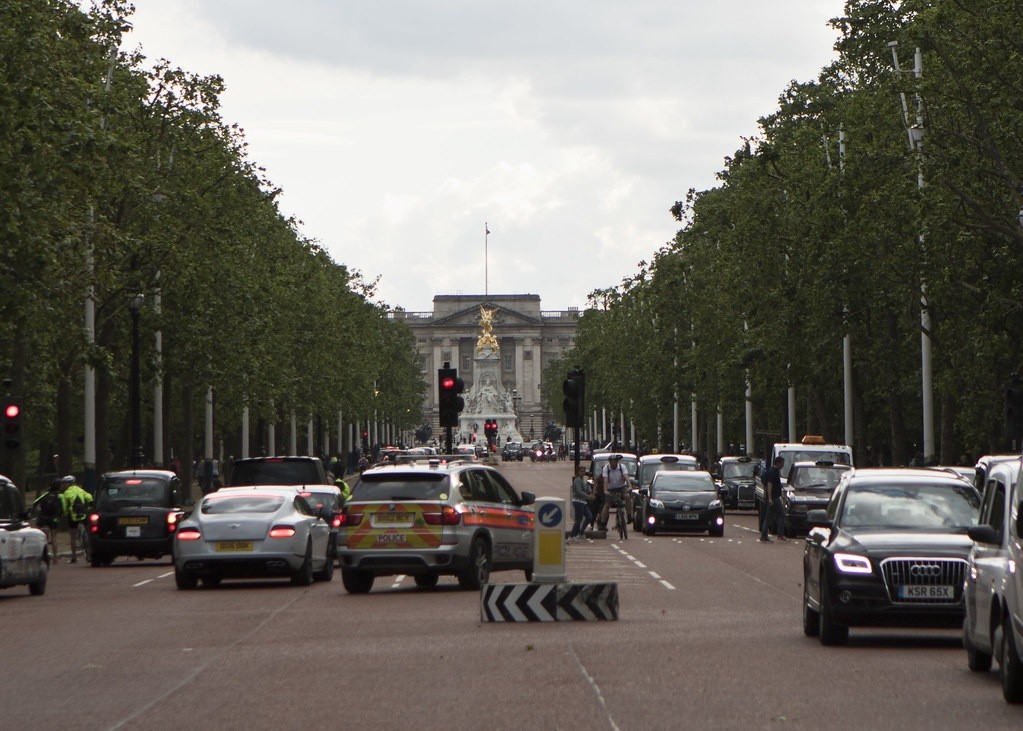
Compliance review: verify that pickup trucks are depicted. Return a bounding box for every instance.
[754,435,855,521]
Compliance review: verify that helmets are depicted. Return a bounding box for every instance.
[51,478,63,486]
[609,455,618,462]
[62,474,77,483]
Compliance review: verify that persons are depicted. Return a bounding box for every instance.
[169,453,237,492]
[323,445,380,479]
[40,475,93,564]
[428,448,458,459]
[558,444,566,461]
[572,455,635,535]
[825,472,836,487]
[760,457,791,542]
[796,468,810,486]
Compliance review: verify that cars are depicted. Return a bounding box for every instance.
[802,466,987,648]
[171,485,333,588]
[83,469,183,570]
[230,455,328,486]
[591,453,697,505]
[0,474,53,593]
[781,459,851,536]
[719,457,769,507]
[643,469,726,534]
[374,441,593,464]
[338,458,541,588]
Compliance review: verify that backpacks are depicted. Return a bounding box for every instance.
[40,492,59,517]
[66,487,85,514]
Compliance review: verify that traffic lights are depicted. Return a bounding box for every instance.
[491,420,498,437]
[563,370,584,427]
[484,419,491,436]
[1,393,22,450]
[438,367,465,427]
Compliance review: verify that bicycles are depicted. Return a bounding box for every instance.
[611,494,631,539]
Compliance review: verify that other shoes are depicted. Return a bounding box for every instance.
[760,538,773,543]
[567,535,591,542]
[627,515,635,524]
[777,536,791,542]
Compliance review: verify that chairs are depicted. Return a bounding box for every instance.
[853,502,881,526]
